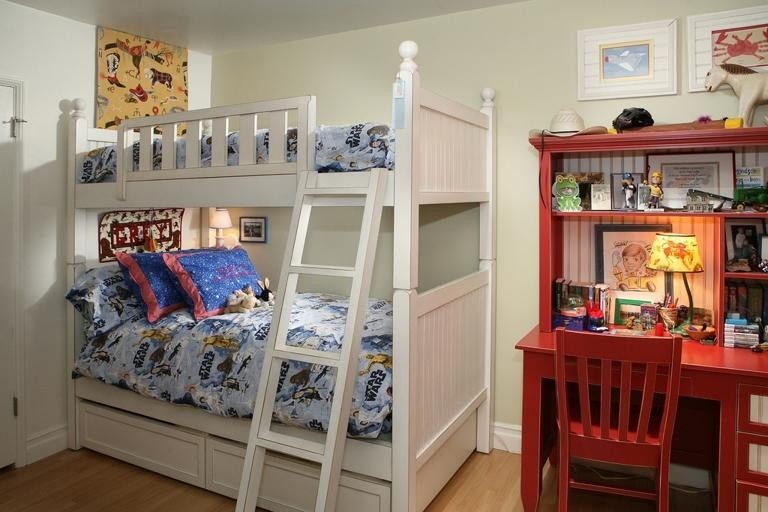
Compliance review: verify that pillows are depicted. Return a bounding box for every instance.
[113,251,190,324]
[161,243,264,322]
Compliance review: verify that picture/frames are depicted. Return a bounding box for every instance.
[725,217,766,264]
[576,18,678,102]
[239,216,267,243]
[686,4,767,94]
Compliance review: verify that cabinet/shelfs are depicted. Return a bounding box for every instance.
[513,128,766,511]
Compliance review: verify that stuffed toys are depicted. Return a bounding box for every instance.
[223,284,261,314]
[253,276,275,307]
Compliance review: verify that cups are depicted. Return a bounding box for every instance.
[660,307,678,329]
[656,323,663,337]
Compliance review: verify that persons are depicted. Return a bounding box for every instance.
[643,170,664,209]
[620,171,637,210]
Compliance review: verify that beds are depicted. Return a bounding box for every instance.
[65,41,497,512]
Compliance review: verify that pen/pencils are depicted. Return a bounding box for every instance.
[658,293,679,308]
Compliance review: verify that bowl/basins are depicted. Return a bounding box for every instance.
[682,325,716,340]
[550,109,584,132]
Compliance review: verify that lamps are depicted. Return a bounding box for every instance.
[644,231,705,337]
[208,209,232,247]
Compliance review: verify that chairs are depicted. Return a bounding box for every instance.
[554,325,682,512]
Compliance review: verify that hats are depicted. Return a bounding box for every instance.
[529,110,608,139]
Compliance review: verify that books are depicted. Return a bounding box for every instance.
[554,277,611,326]
[723,312,760,349]
